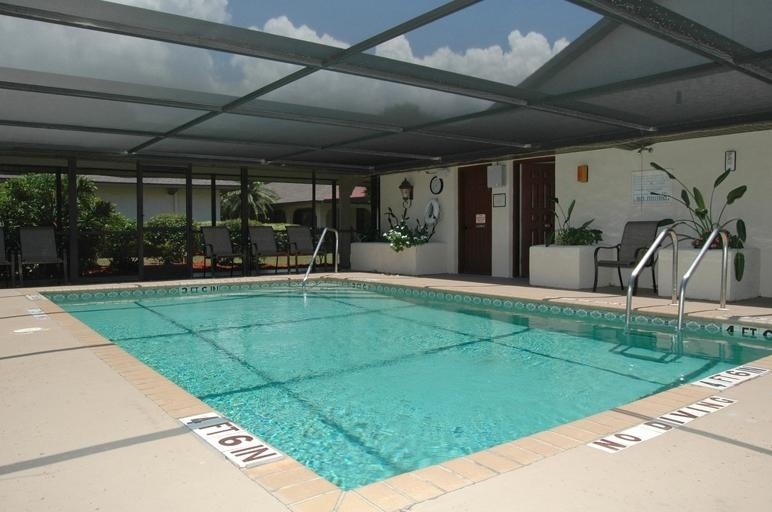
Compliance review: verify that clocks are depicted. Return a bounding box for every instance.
[429,175,443,195]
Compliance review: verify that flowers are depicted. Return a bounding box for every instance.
[382,207,437,253]
[646,150,752,283]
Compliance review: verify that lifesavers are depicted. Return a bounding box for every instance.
[424,199,439,225]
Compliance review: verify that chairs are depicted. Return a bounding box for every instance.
[0,225,70,289]
[199,223,327,279]
[592,218,668,296]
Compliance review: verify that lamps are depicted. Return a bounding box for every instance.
[398,178,414,200]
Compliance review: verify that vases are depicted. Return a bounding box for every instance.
[350,240,446,277]
[656,245,759,302]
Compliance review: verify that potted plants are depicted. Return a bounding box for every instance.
[526,193,612,292]
